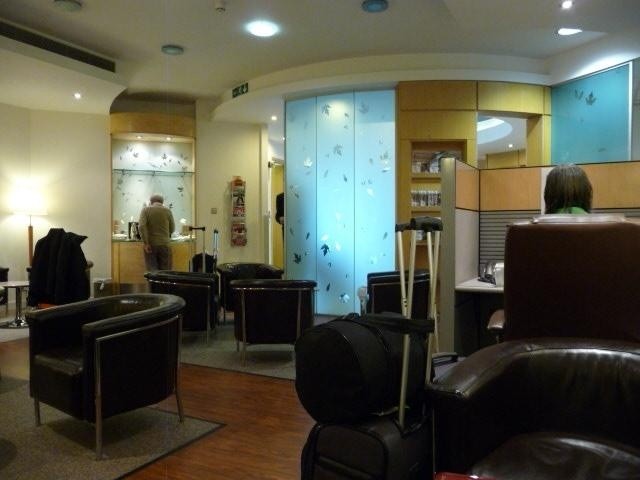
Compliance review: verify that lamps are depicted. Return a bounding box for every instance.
[13,173,48,267]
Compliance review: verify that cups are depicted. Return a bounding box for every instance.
[478,262,495,281]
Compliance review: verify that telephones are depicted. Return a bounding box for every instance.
[481,261,495,283]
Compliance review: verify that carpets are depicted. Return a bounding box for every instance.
[178,312,338,383]
[0,374,227,480]
[0,321,28,345]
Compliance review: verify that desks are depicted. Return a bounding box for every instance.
[1,280,32,328]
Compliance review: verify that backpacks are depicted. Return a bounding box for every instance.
[27,228,90,306]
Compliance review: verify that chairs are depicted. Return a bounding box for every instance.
[24,293,186,462]
[488,217,639,342]
[216,262,284,325]
[424,334,640,480]
[232,278,317,368]
[145,273,219,344]
[1,267,11,314]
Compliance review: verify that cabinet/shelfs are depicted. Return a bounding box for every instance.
[111,112,196,286]
[480,83,552,115]
[398,80,477,316]
[524,117,553,166]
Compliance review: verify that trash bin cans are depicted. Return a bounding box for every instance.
[94,278,112,298]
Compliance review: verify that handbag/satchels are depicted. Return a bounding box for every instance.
[295,311,428,425]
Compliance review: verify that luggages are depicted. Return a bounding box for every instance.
[302,216,441,479]
[189,230,218,273]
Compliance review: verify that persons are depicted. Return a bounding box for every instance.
[542,160,592,214]
[138,195,175,271]
[275,179,284,242]
[232,176,246,246]
